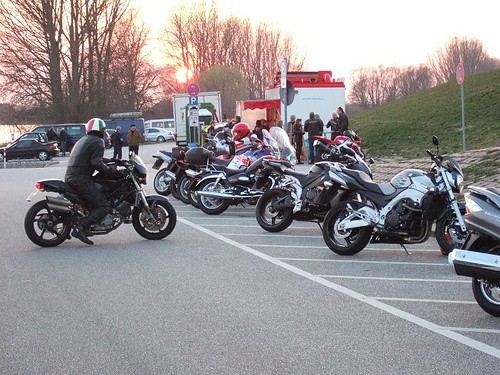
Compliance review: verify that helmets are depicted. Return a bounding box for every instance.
[86,118,106,139]
[232,122,250,141]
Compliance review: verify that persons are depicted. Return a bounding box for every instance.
[126,124,142,159]
[46,128,57,142]
[111,126,126,159]
[65,118,114,245]
[326,107,348,140]
[226,116,241,129]
[60,128,67,156]
[208,122,215,136]
[304,112,323,164]
[254,119,270,140]
[288,115,306,164]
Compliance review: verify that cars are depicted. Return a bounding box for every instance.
[145,127,174,143]
[0,132,60,163]
[104,128,124,149]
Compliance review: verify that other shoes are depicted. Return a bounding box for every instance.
[308,161,314,164]
[71,228,94,245]
[298,162,304,164]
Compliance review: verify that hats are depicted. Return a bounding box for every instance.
[291,115,295,119]
[116,126,121,131]
[131,124,136,126]
[314,114,319,116]
[310,112,314,117]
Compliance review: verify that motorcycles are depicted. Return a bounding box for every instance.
[23,150,178,248]
[149,129,472,256]
[447,184,500,318]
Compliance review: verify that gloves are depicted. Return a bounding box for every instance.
[109,168,124,179]
[110,157,123,166]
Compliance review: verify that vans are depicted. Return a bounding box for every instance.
[30,123,88,147]
[143,118,177,135]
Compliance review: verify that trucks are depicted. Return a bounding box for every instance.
[103,112,145,143]
